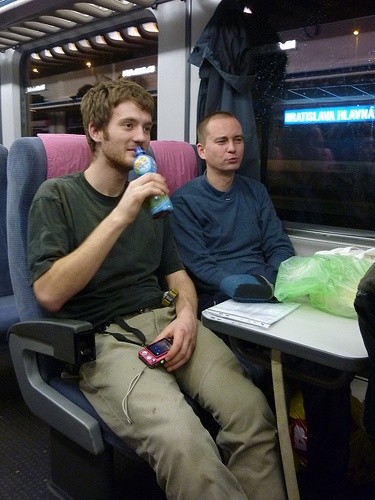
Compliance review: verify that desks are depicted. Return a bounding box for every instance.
[201,300,368,500]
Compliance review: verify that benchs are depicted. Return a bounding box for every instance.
[7,134,202,500]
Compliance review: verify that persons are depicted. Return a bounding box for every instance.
[27,80,290,500]
[168,112,353,500]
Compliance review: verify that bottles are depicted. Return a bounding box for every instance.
[133,147,174,218]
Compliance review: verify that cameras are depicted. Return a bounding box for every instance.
[138,337,172,369]
